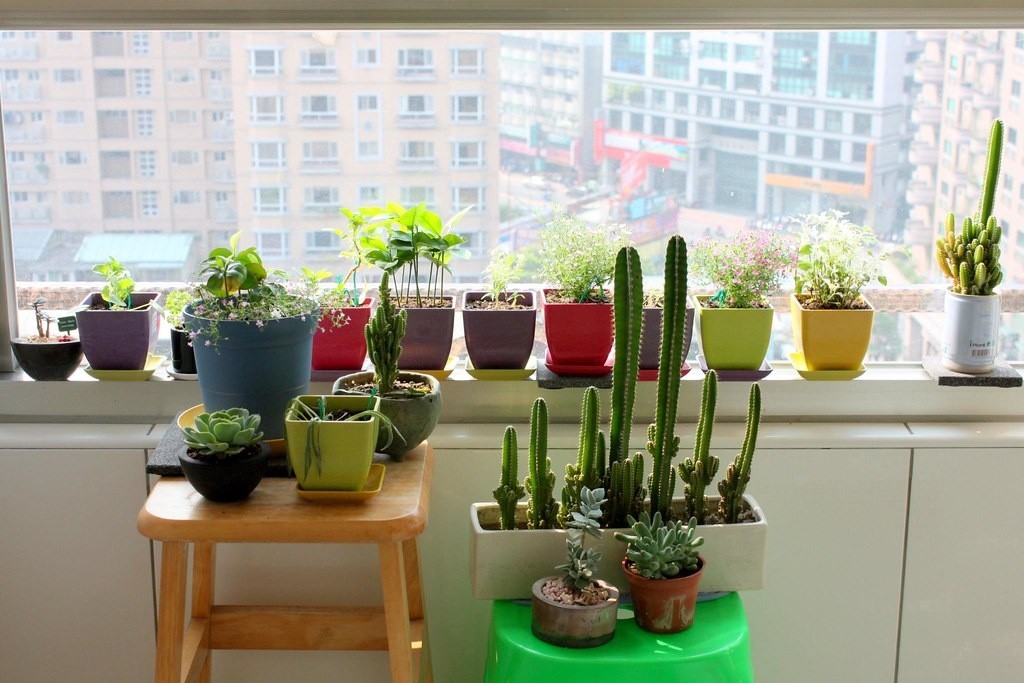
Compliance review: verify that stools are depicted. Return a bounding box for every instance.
[482,593,754,683]
[137,438,442,683]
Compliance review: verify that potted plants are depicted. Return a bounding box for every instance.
[609,280,697,373]
[10,298,83,383]
[465,240,768,598]
[360,197,470,372]
[283,388,405,491]
[332,268,442,456]
[618,506,712,640]
[75,256,161,371]
[159,287,206,372]
[935,117,1004,372]
[462,246,536,370]
[176,407,270,504]
[529,470,615,648]
[298,197,384,371]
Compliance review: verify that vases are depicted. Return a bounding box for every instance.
[792,282,873,368]
[544,287,618,370]
[183,294,321,437]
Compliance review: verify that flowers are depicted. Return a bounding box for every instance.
[688,221,795,308]
[173,235,345,344]
[536,218,628,305]
[778,208,910,304]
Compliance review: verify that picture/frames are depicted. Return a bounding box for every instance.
[697,298,772,375]
